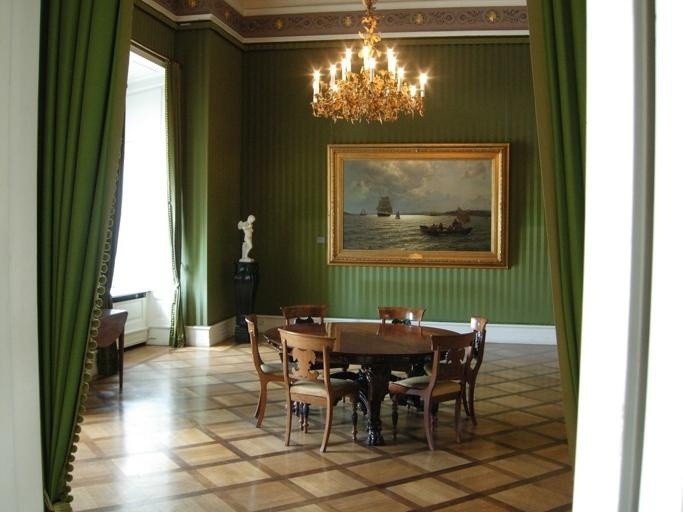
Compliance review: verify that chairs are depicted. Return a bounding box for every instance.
[245,314,304,430]
[279,304,349,403]
[378,306,426,408]
[424,315,489,425]
[388,330,477,451]
[277,327,360,453]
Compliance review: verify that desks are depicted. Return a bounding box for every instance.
[264,322,464,445]
[97,309,128,392]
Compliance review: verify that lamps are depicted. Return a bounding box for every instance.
[310,0,427,125]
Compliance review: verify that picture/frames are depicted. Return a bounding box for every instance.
[326,143,510,268]
